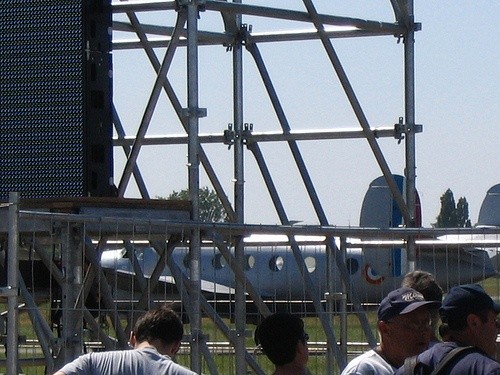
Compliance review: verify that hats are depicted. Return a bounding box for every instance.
[377,288,440,321]
[439,284,500,325]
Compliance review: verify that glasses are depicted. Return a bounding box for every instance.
[386,317,436,331]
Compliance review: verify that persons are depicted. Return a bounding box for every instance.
[51,307,201,375]
[340,270,500,375]
[252,313,318,375]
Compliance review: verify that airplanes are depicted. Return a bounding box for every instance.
[99,174,500,316]
